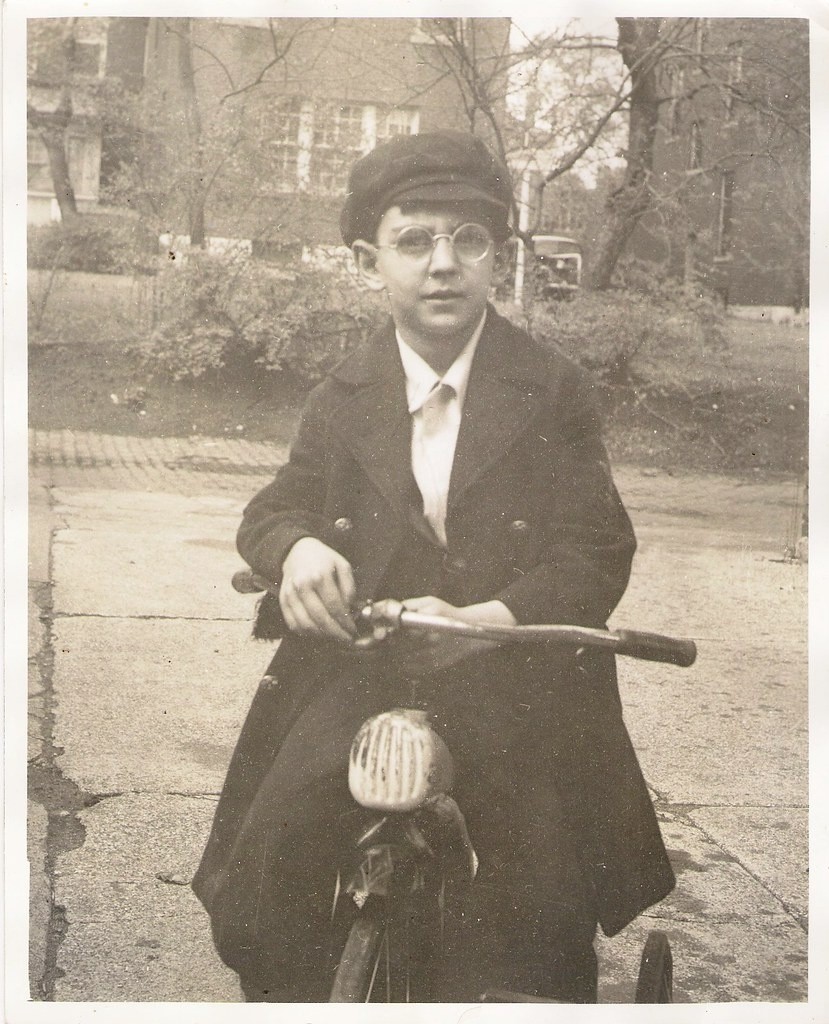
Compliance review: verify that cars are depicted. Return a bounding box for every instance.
[41,207,163,276]
[497,235,585,303]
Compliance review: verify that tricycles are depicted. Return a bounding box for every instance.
[232,569,698,1003]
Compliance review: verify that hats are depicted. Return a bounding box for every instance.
[339,129,512,248]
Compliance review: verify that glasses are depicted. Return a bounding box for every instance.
[371,222,497,265]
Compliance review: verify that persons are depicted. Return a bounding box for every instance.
[188,130,674,1003]
